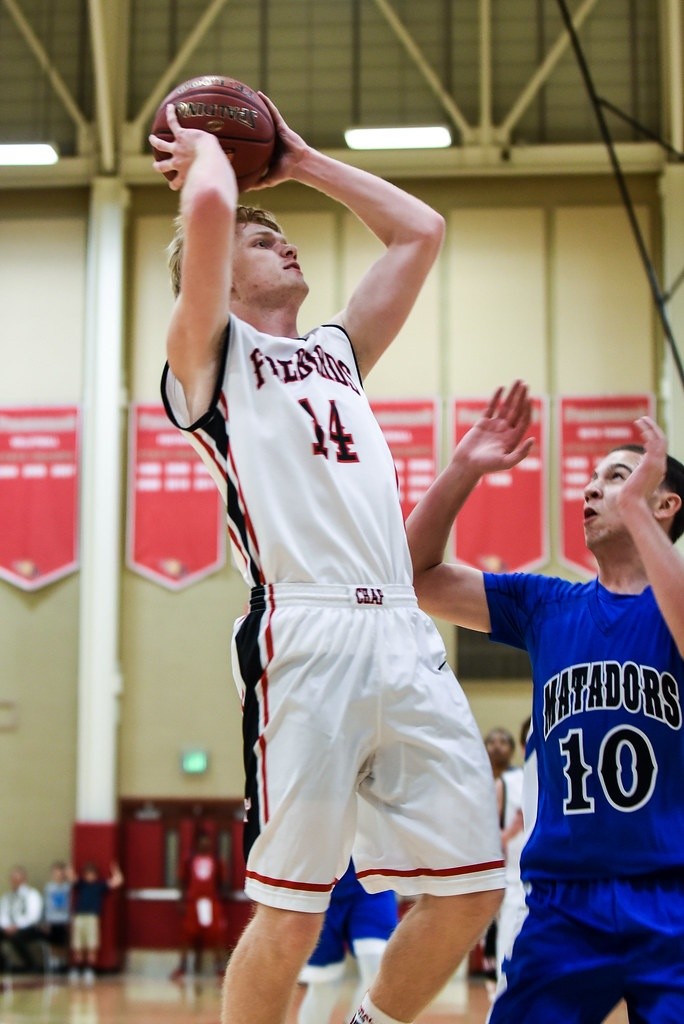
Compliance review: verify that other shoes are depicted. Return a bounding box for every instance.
[84,971,95,986]
[68,969,82,984]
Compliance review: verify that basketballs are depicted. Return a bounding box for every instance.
[151,77,279,196]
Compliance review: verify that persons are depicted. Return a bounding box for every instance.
[284,713,529,1024]
[165,818,231,979]
[64,861,123,985]
[402,377,684,1024]
[0,859,73,975]
[148,90,504,1024]
